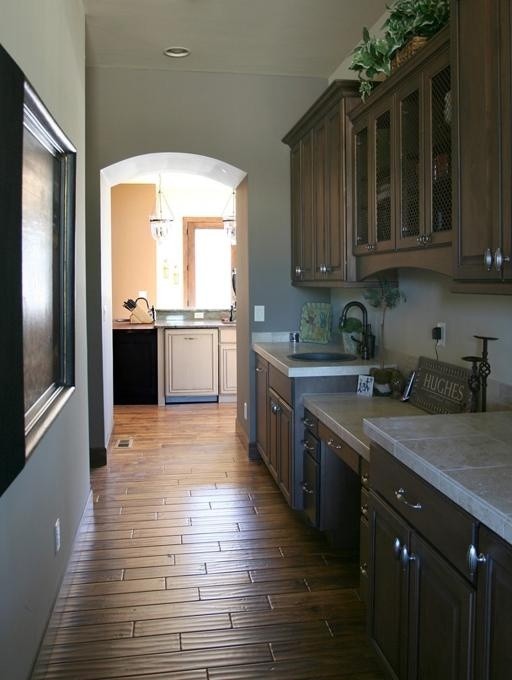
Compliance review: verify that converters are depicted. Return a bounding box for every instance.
[431,327,442,340]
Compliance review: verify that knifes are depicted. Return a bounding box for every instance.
[122,298,136,312]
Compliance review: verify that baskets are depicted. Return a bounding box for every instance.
[390,34,429,74]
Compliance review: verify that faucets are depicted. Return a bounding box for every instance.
[339,301,376,358]
[134,298,156,321]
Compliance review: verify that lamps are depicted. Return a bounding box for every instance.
[223,186,238,246]
[150,172,175,242]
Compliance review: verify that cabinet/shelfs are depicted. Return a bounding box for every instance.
[351,442,512,680]
[219,327,236,403]
[163,327,218,406]
[301,390,459,607]
[345,1,509,300]
[281,77,395,289]
[253,354,397,510]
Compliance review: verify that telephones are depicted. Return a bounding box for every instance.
[399,370,416,402]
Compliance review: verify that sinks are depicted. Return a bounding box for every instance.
[287,352,358,362]
[115,317,132,322]
